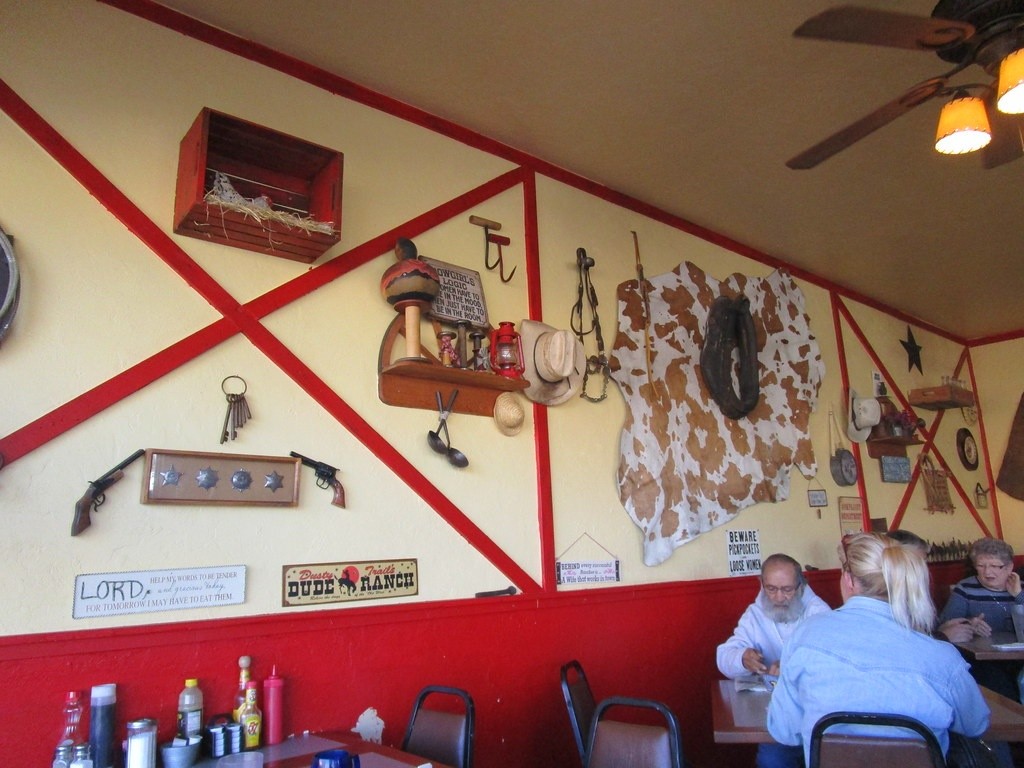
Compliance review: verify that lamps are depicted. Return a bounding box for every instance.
[934,87,992,155]
[489,322,526,378]
[996,47,1024,114]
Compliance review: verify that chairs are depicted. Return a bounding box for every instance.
[401,657,699,768]
[809,711,948,768]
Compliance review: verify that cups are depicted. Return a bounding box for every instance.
[1009,604,1024,645]
[310,749,361,768]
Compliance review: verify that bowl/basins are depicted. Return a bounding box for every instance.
[160,738,202,768]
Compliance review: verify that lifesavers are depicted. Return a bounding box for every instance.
[699,295,760,421]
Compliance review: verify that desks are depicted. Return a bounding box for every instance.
[157,729,459,768]
[710,679,1024,744]
[953,631,1024,661]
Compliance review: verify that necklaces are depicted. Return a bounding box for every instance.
[990,594,1006,611]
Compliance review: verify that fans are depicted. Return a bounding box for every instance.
[785,0,1024,171]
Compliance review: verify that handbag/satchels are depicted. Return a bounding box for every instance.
[947,732,998,768]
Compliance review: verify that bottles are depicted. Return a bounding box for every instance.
[262,664,286,746]
[233,656,254,727]
[225,722,244,754]
[207,725,227,758]
[216,750,264,768]
[178,678,203,742]
[940,375,968,391]
[52,691,95,768]
[126,717,158,768]
[88,683,118,768]
[239,681,263,751]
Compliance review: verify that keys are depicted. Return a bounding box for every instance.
[219,392,251,444]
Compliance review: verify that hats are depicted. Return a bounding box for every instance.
[848,387,880,443]
[494,392,526,436]
[517,319,586,405]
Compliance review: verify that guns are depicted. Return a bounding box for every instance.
[289,450,347,510]
[69,447,145,537]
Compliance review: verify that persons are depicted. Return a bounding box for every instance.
[937,536,1024,768]
[885,529,992,644]
[767,530,991,768]
[715,554,834,768]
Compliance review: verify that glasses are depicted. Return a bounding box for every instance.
[974,562,1008,573]
[762,579,801,595]
[842,533,859,586]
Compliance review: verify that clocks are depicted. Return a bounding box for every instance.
[956,428,978,471]
